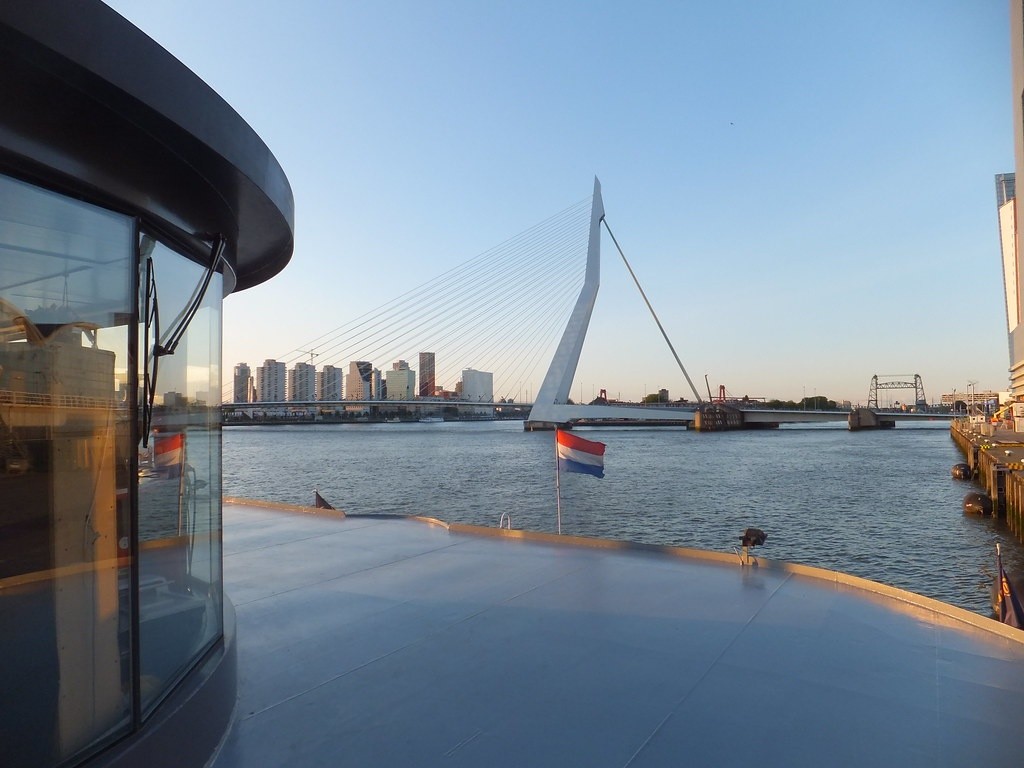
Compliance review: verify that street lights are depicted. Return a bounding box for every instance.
[953,388,958,420]
[966,383,972,415]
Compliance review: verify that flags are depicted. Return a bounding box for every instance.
[153,434,184,477]
[557,429,605,479]
[996,559,1024,629]
